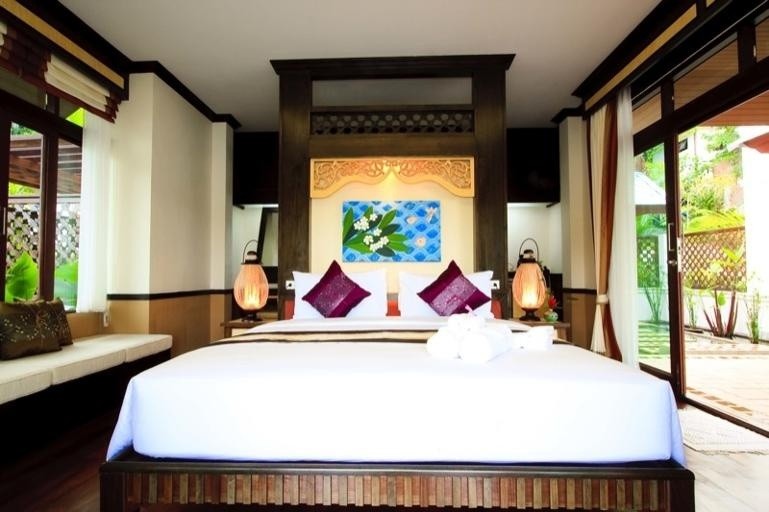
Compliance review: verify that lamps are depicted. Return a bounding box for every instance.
[233,239,270,322]
[512,237,549,321]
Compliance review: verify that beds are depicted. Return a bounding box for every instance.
[98,294,697,511]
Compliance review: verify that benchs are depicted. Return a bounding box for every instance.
[0,309,174,464]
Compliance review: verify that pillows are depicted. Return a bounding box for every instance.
[1,296,73,361]
[291,260,495,325]
